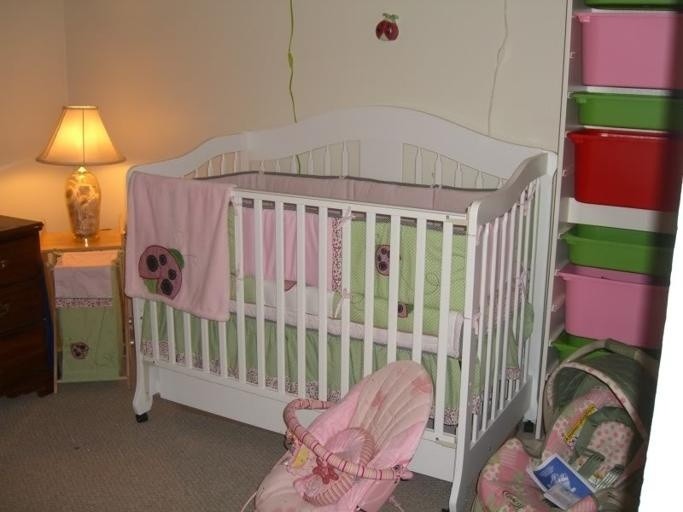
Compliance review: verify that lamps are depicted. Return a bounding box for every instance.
[36,105,124,244]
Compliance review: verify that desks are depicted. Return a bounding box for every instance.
[48,247,130,384]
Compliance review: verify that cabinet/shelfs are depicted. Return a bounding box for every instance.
[0,215,60,398]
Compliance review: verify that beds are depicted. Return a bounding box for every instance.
[126,105,559,512]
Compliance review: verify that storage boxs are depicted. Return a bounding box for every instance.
[563,197,678,234]
[563,124,682,211]
[553,262,669,353]
[570,83,681,131]
[572,0,682,90]
[563,226,673,279]
